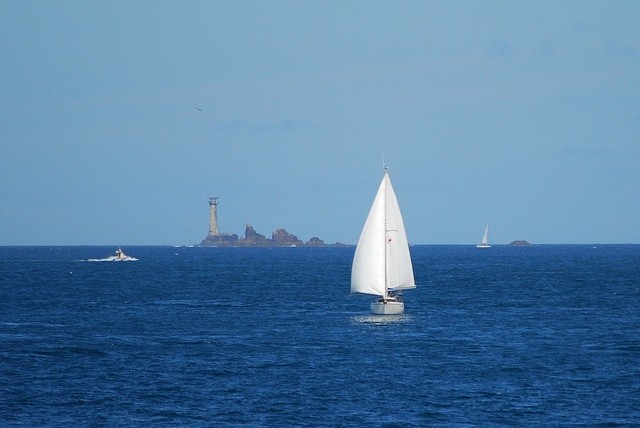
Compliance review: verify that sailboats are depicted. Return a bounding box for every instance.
[349,172,417,315]
[477,225,492,248]
